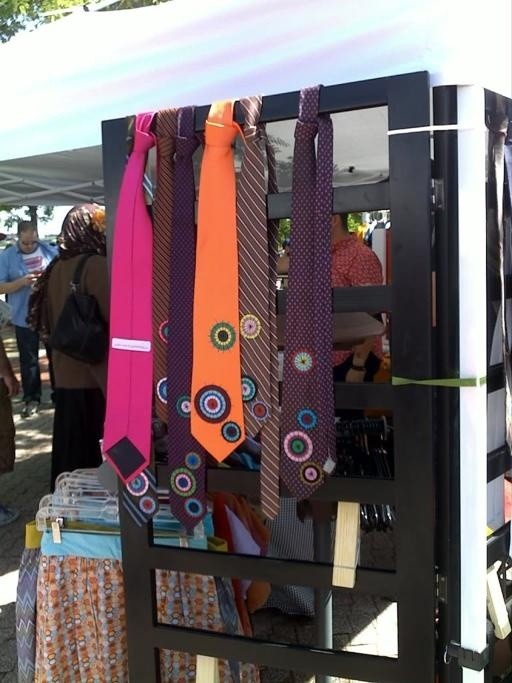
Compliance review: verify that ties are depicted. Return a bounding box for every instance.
[278,83,338,502]
[118,113,156,528]
[152,107,176,424]
[190,101,246,464]
[236,95,278,521]
[168,107,207,530]
[102,110,156,485]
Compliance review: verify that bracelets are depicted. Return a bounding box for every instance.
[350,364,366,372]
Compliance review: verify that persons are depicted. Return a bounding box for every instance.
[276,211,386,422]
[22,201,113,496]
[0,218,61,417]
[0,336,22,530]
[279,236,292,288]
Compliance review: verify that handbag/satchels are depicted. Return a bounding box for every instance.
[48,255,110,366]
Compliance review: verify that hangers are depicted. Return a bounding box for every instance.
[32,440,206,548]
[334,413,396,532]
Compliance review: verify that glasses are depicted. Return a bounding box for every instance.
[22,240,36,246]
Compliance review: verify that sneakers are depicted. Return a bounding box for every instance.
[0,503,22,527]
[20,399,41,418]
